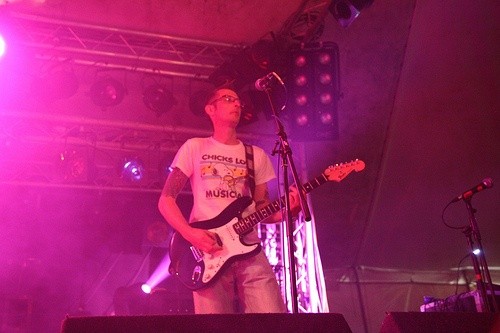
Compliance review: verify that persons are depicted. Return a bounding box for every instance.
[157,80,307,314]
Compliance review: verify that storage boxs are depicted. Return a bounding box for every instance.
[419,288,500,312]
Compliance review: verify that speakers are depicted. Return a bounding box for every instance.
[62,313,352,333]
[378,311,500,333]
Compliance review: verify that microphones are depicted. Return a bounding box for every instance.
[255,72,275,90]
[452,178,494,203]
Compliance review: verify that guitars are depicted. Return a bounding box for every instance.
[168,158,366,292]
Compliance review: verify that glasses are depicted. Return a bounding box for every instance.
[210,95,244,108]
